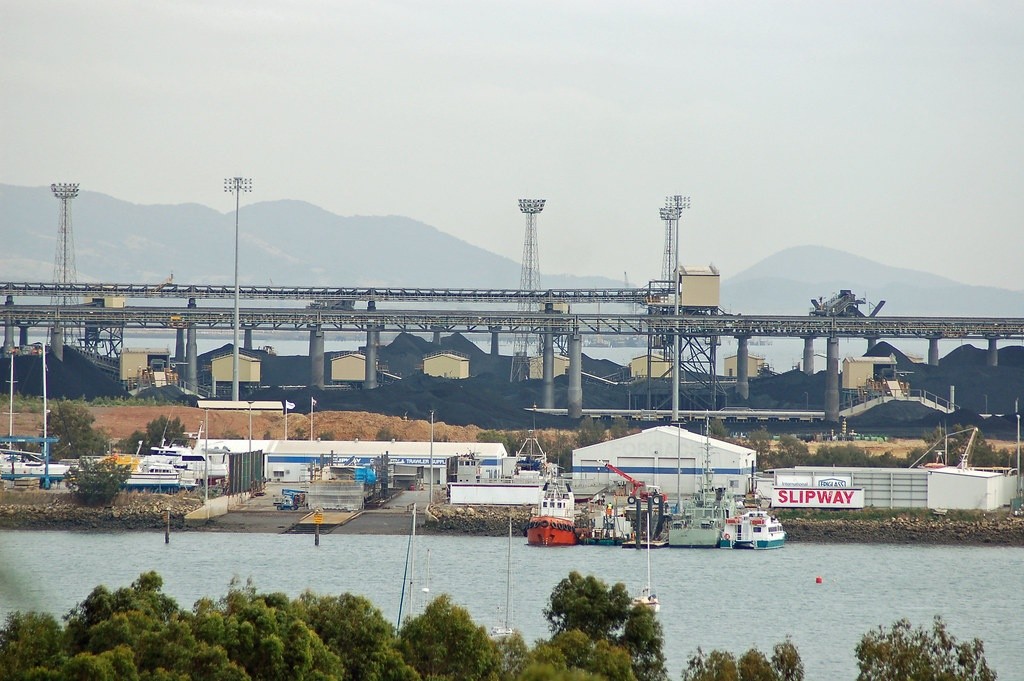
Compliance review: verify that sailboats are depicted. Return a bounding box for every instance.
[627,511,661,617]
[483,516,516,647]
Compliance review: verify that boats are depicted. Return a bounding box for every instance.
[836,367,959,429]
[523,468,582,545]
[0,421,230,494]
[574,469,788,550]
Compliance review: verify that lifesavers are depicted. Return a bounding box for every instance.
[724,534,730,540]
[541,520,549,527]
[568,525,572,531]
[526,521,529,529]
[534,521,539,528]
[558,522,564,530]
[529,522,534,528]
[550,521,557,529]
[564,523,568,530]
[522,526,527,537]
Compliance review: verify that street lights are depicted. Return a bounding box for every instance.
[663,195,693,423]
[518,196,546,323]
[803,391,809,413]
[223,176,254,400]
[50,178,81,346]
[982,393,989,414]
[660,207,683,302]
